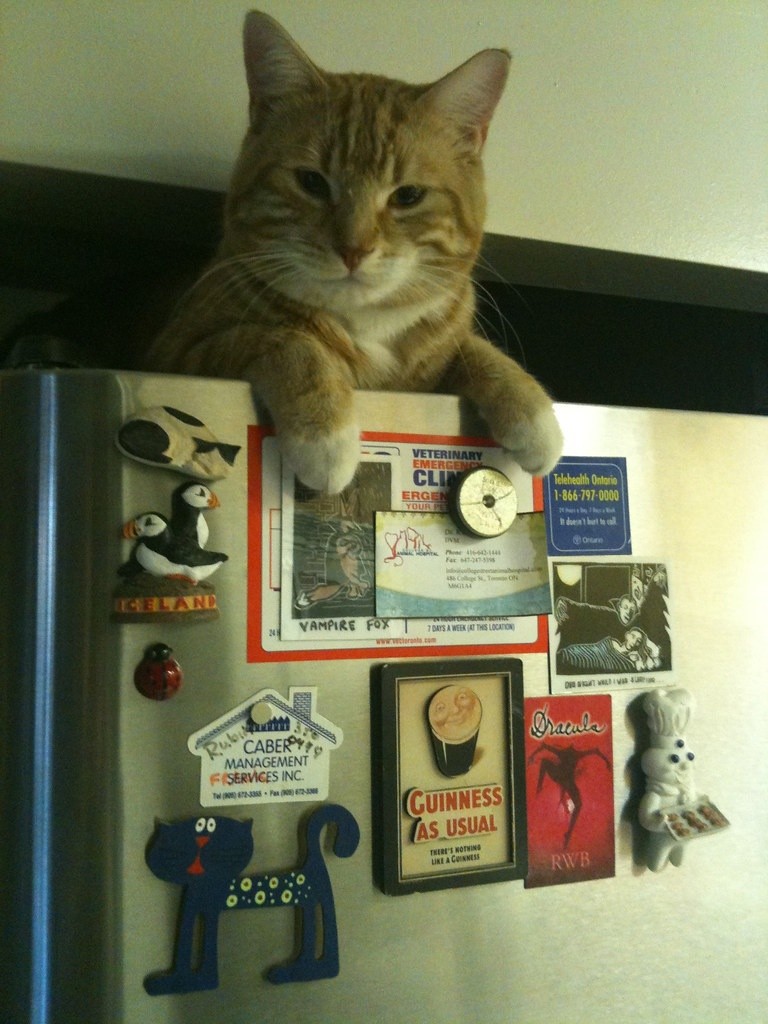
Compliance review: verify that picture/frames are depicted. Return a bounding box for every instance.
[370,658,526,895]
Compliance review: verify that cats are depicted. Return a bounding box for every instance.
[134,9,568,498]
